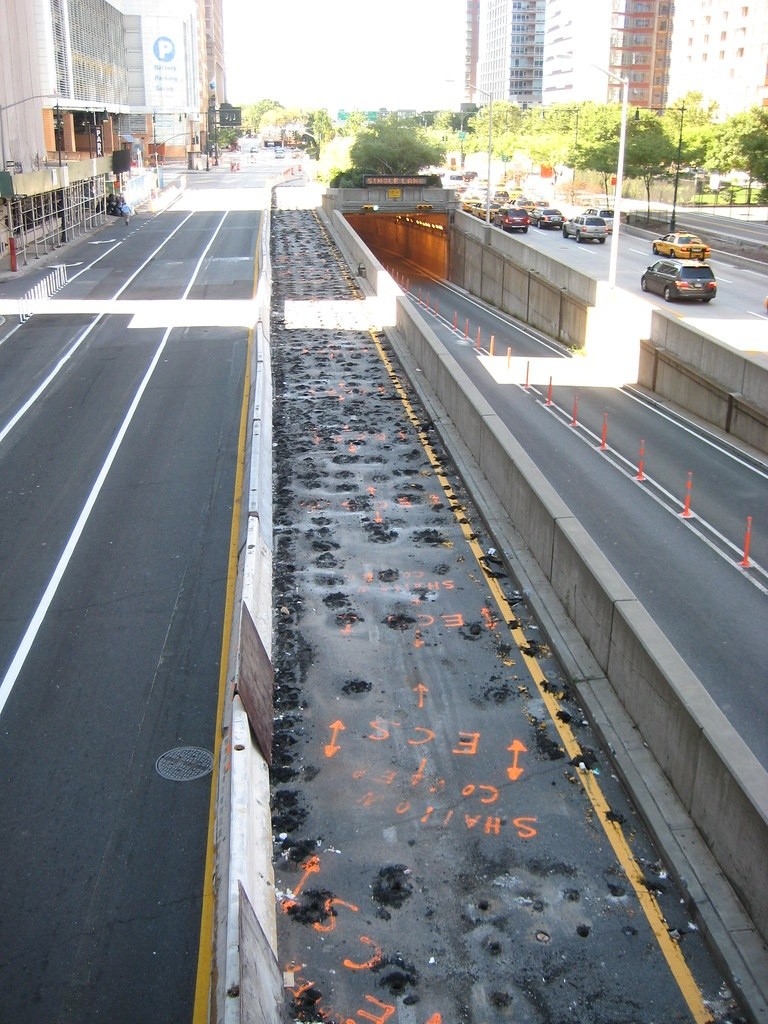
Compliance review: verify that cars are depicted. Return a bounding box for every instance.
[580,207,615,236]
[562,215,608,244]
[449,170,550,222]
[527,208,567,230]
[640,258,717,303]
[652,230,711,263]
[250,145,301,163]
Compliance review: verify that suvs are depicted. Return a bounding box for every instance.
[492,206,530,233]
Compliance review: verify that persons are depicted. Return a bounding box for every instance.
[121,202,131,226]
[625,212,630,224]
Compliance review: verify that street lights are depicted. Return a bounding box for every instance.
[633,101,687,232]
[558,45,630,289]
[0,93,61,271]
[539,107,580,208]
[154,133,190,189]
[446,78,494,245]
[53,105,110,243]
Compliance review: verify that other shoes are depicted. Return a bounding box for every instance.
[126,222,128,226]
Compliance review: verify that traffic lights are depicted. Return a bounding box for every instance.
[361,205,380,212]
[415,204,434,211]
